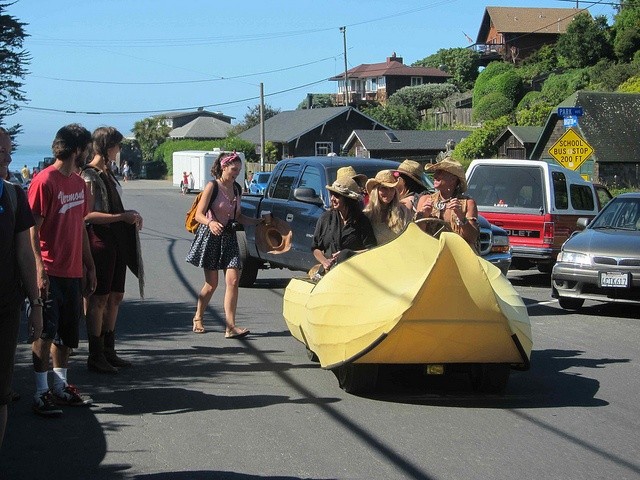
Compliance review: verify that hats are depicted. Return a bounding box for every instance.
[424,158,467,192]
[255,217,292,254]
[390,159,427,189]
[337,166,368,187]
[326,176,364,201]
[365,169,404,196]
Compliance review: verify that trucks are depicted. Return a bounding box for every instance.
[172,150,247,194]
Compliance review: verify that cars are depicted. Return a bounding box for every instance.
[552,192,640,309]
[249,171,272,193]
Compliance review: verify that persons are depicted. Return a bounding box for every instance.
[124,161,129,183]
[0,127,46,457]
[185,150,264,340]
[80,127,143,373]
[182,171,192,195]
[396,158,435,212]
[27,122,97,417]
[338,166,369,205]
[310,177,377,284]
[32,166,36,178]
[21,164,30,179]
[410,158,478,256]
[120,161,124,181]
[361,170,416,246]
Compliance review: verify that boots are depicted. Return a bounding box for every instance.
[104,331,131,367]
[88,331,118,375]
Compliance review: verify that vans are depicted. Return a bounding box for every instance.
[463,159,604,274]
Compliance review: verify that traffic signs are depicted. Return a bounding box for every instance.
[548,129,595,172]
[558,108,584,117]
[563,116,579,127]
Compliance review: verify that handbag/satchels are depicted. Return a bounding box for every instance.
[79,166,115,265]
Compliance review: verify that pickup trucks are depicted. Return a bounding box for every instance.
[236,155,511,288]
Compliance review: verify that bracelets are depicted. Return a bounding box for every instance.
[207,219,214,227]
[30,298,44,307]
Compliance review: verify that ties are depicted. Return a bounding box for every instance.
[339,211,351,226]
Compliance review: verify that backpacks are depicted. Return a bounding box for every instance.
[185,180,242,234]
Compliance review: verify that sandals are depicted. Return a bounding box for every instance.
[193,316,204,332]
[225,326,250,338]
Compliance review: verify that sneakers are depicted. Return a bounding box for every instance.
[51,384,93,405]
[32,387,63,415]
